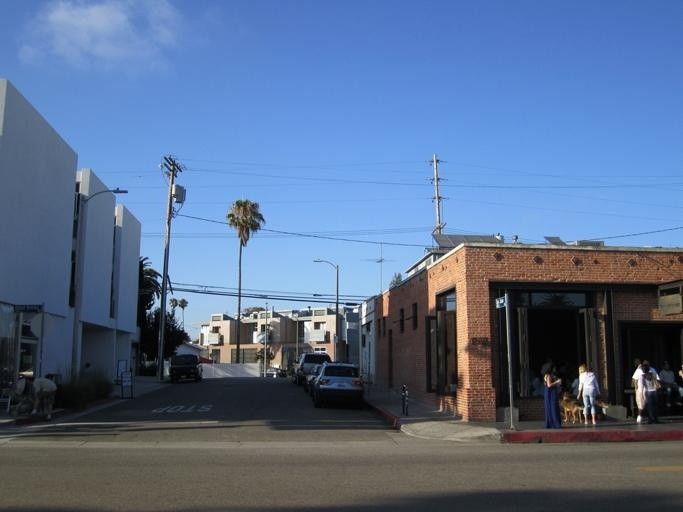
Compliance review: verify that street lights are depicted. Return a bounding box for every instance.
[71,188,128,381]
[313,260,338,362]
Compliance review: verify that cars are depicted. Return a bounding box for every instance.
[168,354,203,383]
[292,353,364,409]
[19,368,33,379]
[260,367,287,378]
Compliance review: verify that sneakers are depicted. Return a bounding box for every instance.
[647,416,661,425]
[638,415,642,424]
[583,418,598,426]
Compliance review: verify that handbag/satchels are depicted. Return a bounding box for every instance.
[652,377,661,390]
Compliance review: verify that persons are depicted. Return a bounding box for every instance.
[27,373,58,421]
[575,363,600,426]
[540,360,562,429]
[568,370,579,396]
[628,357,683,425]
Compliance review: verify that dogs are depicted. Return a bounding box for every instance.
[561,391,584,424]
